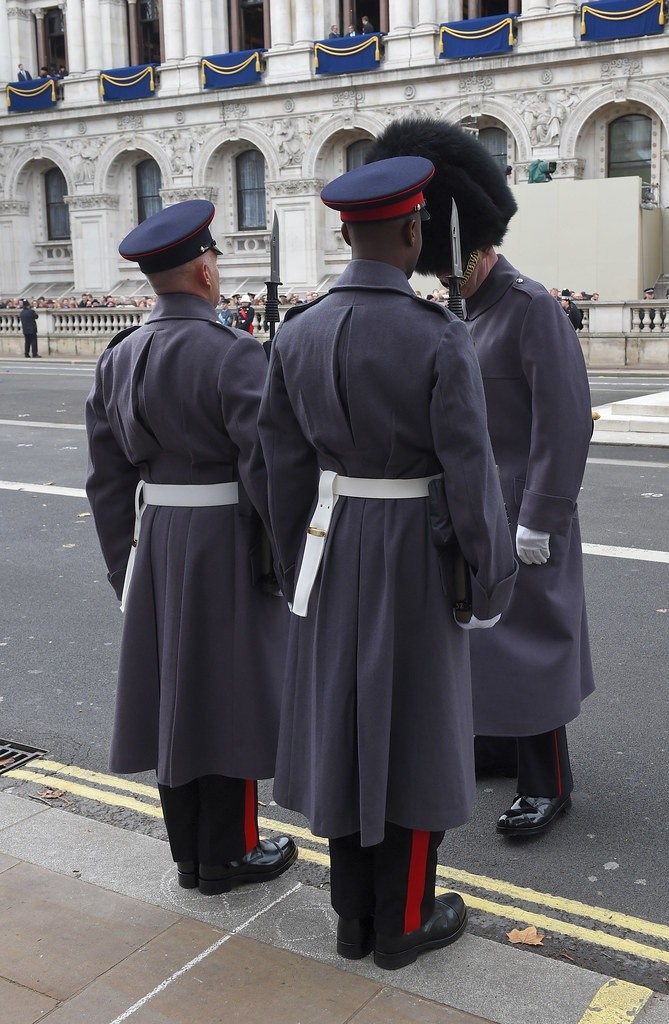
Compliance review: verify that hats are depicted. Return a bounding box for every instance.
[118,200,224,274]
[427,295,433,300]
[666,288,669,296]
[279,295,287,298]
[644,287,654,293]
[561,296,569,300]
[562,289,569,296]
[367,118,519,272]
[23,299,30,306]
[319,156,432,223]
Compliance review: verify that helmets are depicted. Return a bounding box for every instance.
[240,295,251,302]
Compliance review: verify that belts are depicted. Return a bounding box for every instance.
[315,467,441,499]
[142,482,239,508]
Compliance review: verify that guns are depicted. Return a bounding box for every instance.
[260,210,283,361]
[448,196,484,323]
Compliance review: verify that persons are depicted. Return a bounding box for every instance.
[83,200,301,897]
[0,294,158,330]
[216,292,319,338]
[416,287,451,309]
[255,156,520,969]
[550,286,600,334]
[20,300,42,358]
[365,119,597,835]
[639,287,666,332]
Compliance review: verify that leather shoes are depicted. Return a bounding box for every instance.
[177,860,198,889]
[198,835,298,895]
[374,892,468,970]
[338,916,374,960]
[495,793,571,836]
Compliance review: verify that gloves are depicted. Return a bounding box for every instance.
[288,602,292,612]
[517,525,550,564]
[453,605,501,631]
[273,589,283,596]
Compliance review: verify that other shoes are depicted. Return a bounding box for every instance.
[24,352,30,357]
[32,353,42,358]
